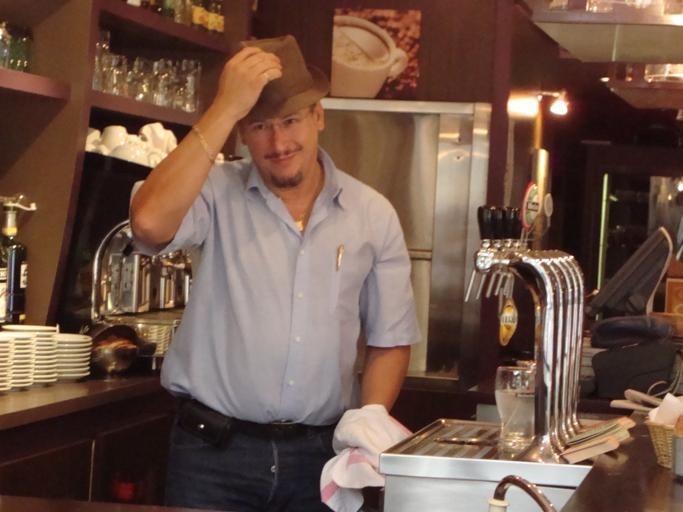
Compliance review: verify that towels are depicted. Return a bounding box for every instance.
[319,403,417,511]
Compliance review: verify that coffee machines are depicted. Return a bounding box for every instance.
[69,199,191,370]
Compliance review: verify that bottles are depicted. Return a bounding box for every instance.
[0,19,33,74]
[515,351,535,391]
[0,191,27,324]
[121,1,225,33]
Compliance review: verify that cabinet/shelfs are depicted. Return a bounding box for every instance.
[1,0,253,325]
[1,390,172,504]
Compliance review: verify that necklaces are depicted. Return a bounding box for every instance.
[293,217,304,233]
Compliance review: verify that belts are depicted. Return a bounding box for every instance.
[172,394,334,444]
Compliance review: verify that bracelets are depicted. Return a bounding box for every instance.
[192,125,215,166]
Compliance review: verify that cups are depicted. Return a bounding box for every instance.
[81,122,181,169]
[90,27,201,112]
[494,364,538,451]
[331,17,412,96]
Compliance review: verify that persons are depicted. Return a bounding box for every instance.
[129,31,422,512]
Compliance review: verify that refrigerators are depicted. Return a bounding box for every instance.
[316,98,496,381]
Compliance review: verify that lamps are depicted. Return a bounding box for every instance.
[539,88,570,115]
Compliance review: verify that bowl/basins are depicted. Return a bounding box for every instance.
[91,343,157,379]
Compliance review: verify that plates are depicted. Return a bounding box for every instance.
[0,322,92,394]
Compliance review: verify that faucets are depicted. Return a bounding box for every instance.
[487,474,558,512]
[462,205,585,466]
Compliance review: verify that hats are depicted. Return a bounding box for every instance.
[231,35,331,125]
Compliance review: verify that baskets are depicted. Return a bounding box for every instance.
[645,417,676,469]
